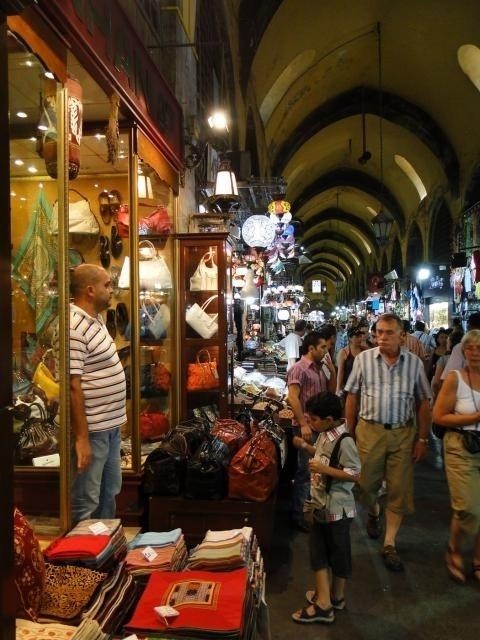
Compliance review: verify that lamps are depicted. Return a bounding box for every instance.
[334,23,407,291]
[242,207,275,257]
[187,112,240,198]
[138,160,154,199]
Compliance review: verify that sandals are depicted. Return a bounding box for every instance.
[291,590,346,624]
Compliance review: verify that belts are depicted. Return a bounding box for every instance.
[362,418,412,431]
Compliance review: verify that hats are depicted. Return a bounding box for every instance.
[348,327,365,337]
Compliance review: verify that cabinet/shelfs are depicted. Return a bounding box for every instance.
[177,233,235,420]
[51,232,172,398]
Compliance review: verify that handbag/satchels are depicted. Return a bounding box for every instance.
[115,203,171,238]
[187,349,218,392]
[52,188,100,236]
[190,246,218,291]
[186,295,219,339]
[118,239,174,289]
[144,417,289,504]
[11,349,62,461]
[124,297,170,443]
[463,431,480,454]
[43,127,81,180]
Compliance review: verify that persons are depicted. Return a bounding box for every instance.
[291,391,363,625]
[61,262,127,521]
[431,329,480,586]
[283,311,480,401]
[341,314,435,574]
[285,331,338,531]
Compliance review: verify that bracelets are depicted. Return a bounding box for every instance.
[300,440,309,450]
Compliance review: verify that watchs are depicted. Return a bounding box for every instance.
[418,438,432,447]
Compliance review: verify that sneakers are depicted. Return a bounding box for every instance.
[381,545,402,570]
[97,190,131,358]
[367,502,384,540]
[444,547,480,584]
